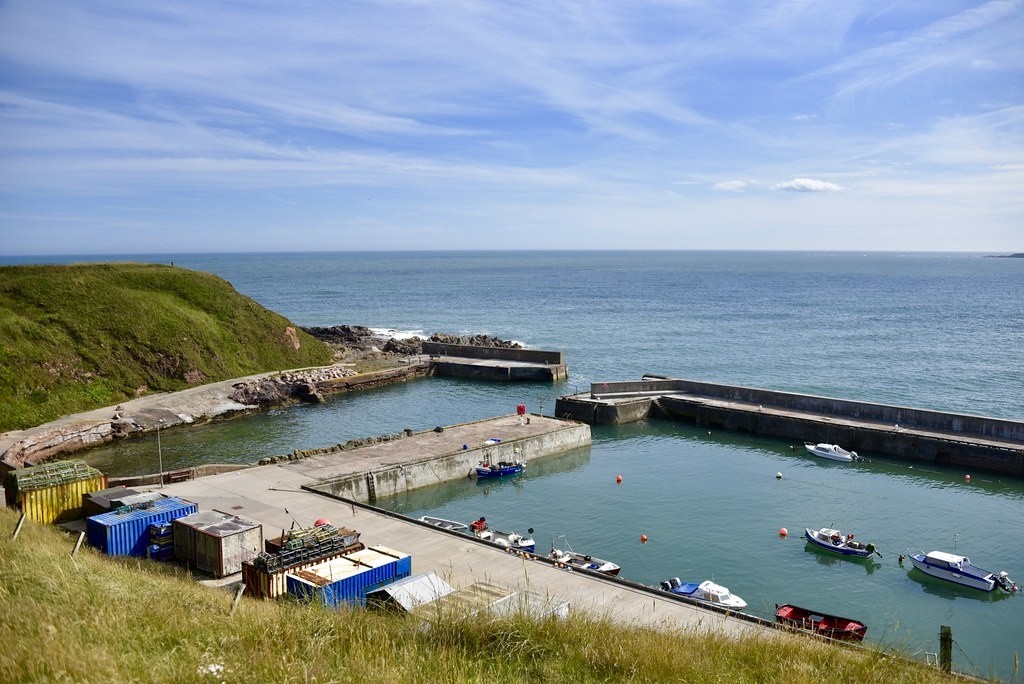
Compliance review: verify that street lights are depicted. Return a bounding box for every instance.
[156,419,164,489]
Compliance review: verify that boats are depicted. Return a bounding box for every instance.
[803,441,865,463]
[656,578,749,612]
[804,522,883,560]
[468,516,536,553]
[417,515,469,533]
[547,532,621,576]
[902,532,1018,595]
[474,451,527,478]
[773,602,868,644]
